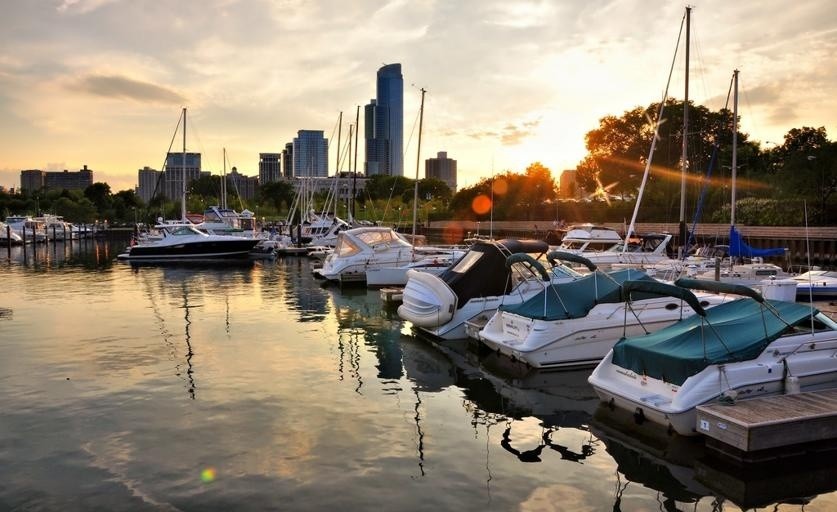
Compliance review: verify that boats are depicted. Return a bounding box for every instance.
[0,210,92,245]
